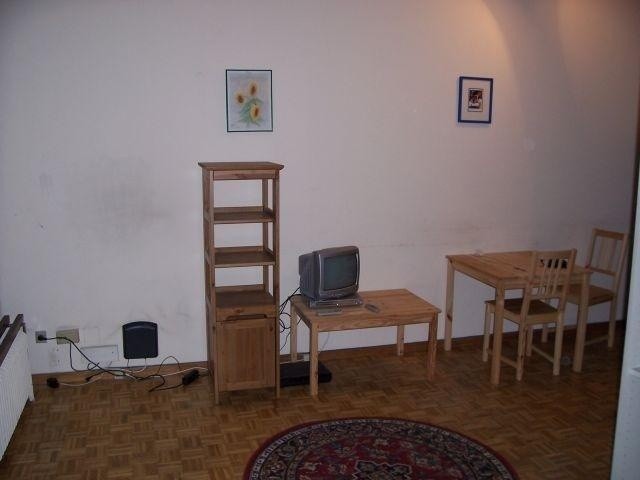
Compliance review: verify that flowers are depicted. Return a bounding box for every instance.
[234,81,263,127]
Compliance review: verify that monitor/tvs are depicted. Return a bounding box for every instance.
[298,246,360,301]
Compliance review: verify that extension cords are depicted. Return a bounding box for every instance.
[183,370,198,385]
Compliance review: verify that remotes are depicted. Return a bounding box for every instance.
[318,310,342,316]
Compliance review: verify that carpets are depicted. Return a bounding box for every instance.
[242,417,520,480]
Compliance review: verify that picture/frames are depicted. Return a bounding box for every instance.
[226,69,273,132]
[458,76,493,123]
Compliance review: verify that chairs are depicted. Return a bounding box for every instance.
[541,228,632,373]
[481,246,577,381]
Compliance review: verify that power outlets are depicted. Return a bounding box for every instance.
[35,331,46,343]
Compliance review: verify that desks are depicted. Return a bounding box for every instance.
[444,249,593,386]
[289,288,442,397]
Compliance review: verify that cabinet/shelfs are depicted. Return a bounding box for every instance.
[199,160,281,404]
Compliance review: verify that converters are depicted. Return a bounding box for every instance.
[47,378,58,388]
